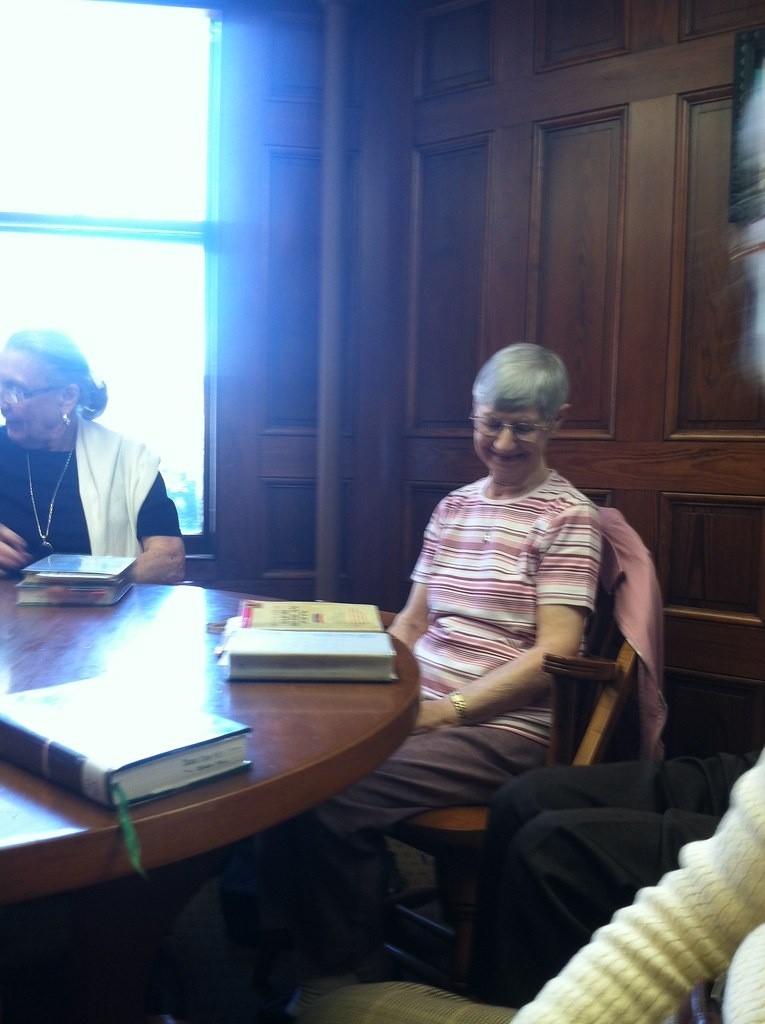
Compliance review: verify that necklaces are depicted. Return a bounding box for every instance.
[481,477,529,546]
[25,432,76,557]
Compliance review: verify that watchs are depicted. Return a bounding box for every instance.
[450,688,470,726]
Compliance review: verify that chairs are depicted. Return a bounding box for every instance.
[382,507,661,996]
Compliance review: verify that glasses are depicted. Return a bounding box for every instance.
[469,408,555,442]
[0,383,62,403]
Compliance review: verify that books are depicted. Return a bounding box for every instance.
[218,597,401,685]
[0,668,254,810]
[14,548,137,611]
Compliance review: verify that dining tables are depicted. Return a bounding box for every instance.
[0,580,419,906]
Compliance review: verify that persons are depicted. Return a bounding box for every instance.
[0,326,188,588]
[484,743,761,999]
[269,343,609,1022]
[286,738,763,1024]
